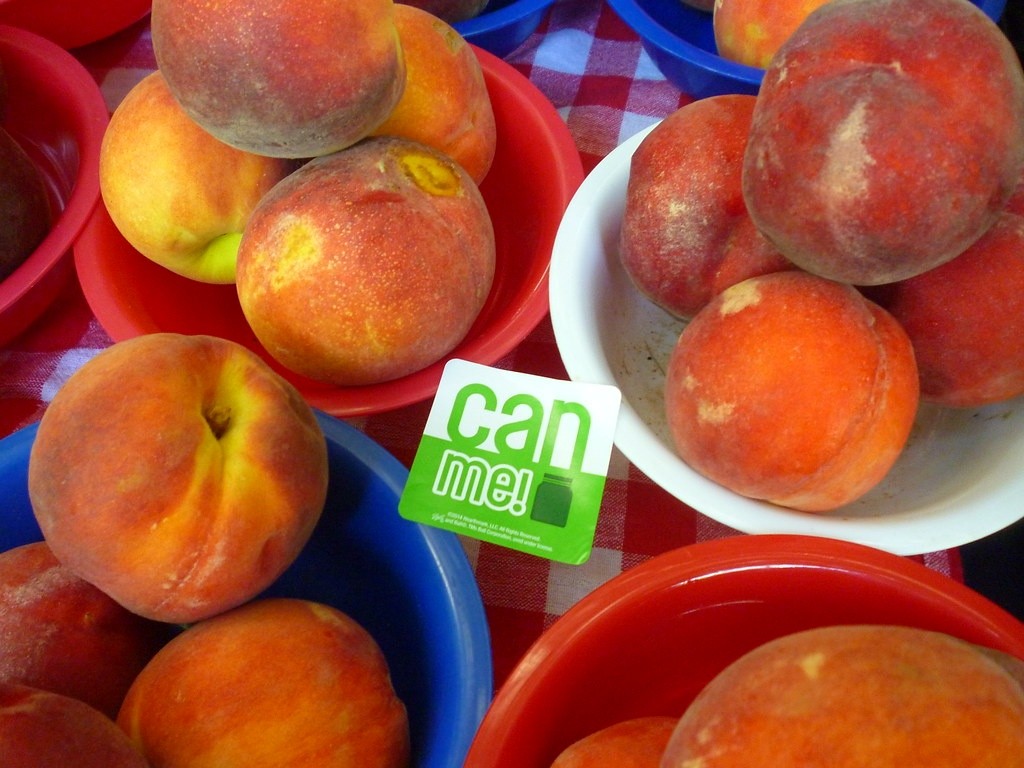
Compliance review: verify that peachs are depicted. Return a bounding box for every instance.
[616,0,1024,513]
[98,0,498,388]
[0,328,408,768]
[546,623,1024,768]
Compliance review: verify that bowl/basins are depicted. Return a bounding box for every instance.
[2,403,495,768]
[467,534,1024,768]
[75,45,584,415]
[610,0,1007,99]
[548,114,1024,558]
[0,24,111,348]
[394,0,555,58]
[0,1,152,51]
